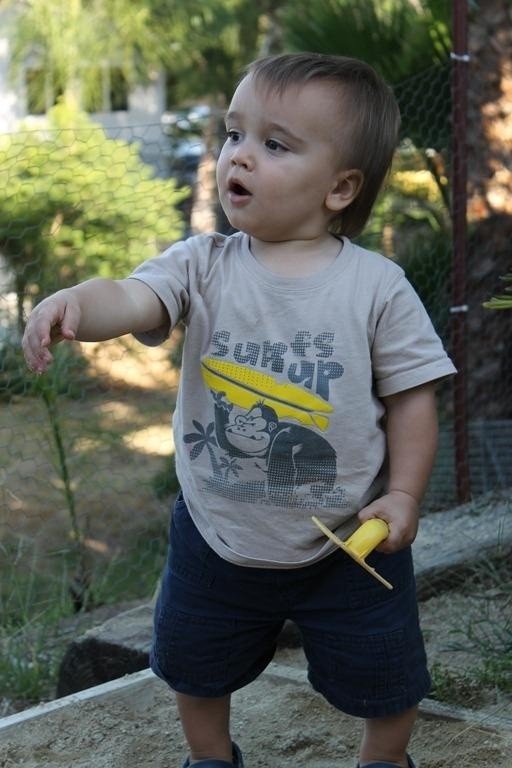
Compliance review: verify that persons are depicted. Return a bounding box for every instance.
[21,53,459,767]
